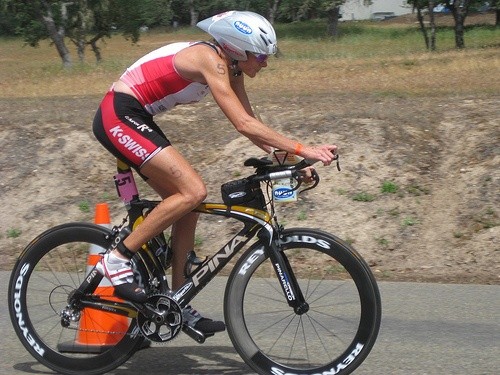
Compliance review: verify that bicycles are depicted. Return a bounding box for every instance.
[5,145,384,375]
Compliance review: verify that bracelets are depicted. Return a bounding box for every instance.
[294,143,304,156]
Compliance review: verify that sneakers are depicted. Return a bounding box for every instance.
[101,251,145,299]
[174,306,226,332]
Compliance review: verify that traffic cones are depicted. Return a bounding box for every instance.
[53,202,152,355]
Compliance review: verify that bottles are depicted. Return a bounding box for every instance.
[149,236,173,270]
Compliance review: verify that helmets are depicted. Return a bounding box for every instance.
[195,10,278,62]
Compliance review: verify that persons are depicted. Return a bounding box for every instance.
[92,10,339,338]
